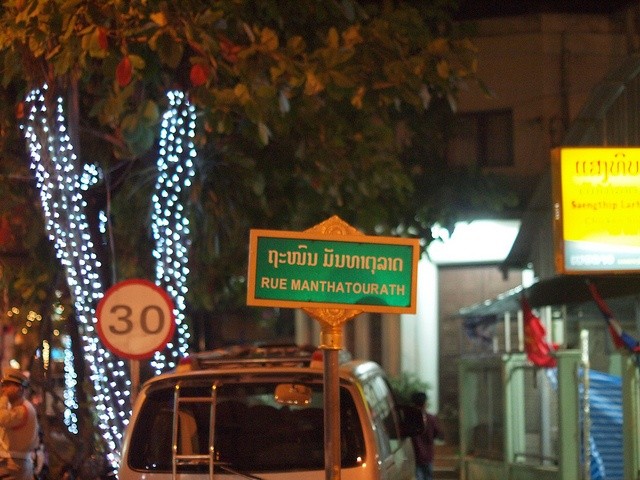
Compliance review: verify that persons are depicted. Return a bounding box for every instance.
[1,367,39,480]
[413,390,444,480]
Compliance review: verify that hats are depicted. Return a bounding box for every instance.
[3,371,28,388]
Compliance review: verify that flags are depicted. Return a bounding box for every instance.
[575,269,638,364]
[513,295,564,388]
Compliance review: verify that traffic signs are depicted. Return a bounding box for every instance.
[95,279,174,361]
[245,227,421,316]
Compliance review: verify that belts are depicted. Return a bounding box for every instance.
[0,451,32,460]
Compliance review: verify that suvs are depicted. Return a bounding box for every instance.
[115,340,426,480]
